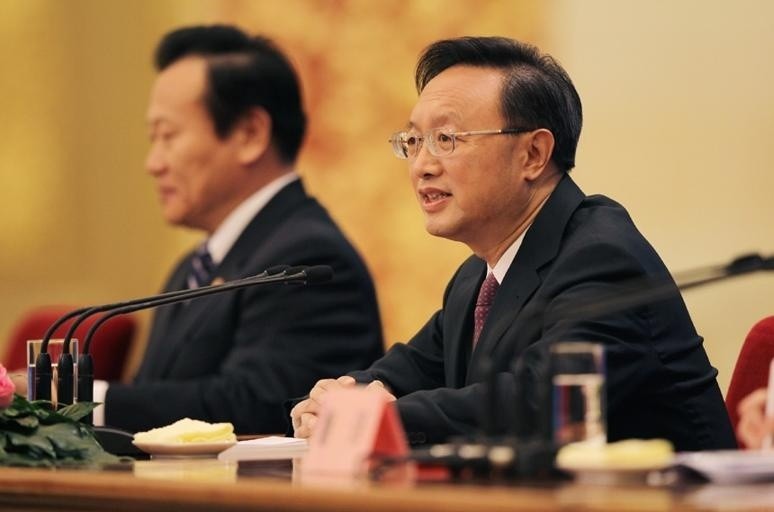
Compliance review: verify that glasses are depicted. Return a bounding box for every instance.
[387,128,535,159]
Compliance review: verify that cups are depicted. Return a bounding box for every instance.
[549,340,607,456]
[26,339,79,408]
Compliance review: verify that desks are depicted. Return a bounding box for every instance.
[0,444,773,512]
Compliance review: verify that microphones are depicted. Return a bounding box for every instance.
[425,253,774,489]
[33,263,334,459]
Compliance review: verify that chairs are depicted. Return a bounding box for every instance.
[721,315,773,446]
[8,308,131,385]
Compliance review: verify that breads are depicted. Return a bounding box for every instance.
[555,437,677,470]
[133,416,237,445]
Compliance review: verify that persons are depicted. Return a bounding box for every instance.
[260,34,740,481]
[735,389,773,450]
[0,20,384,434]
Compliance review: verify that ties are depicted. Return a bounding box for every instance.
[184,252,214,302]
[473,273,496,351]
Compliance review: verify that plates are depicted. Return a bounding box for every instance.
[554,449,773,485]
[129,440,239,458]
